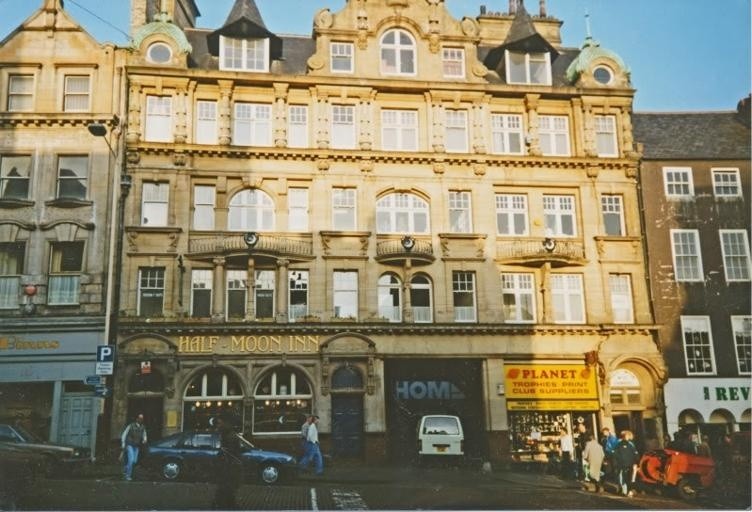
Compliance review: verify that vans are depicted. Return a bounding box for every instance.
[412,414,466,465]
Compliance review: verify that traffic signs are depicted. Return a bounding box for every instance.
[84,376,100,384]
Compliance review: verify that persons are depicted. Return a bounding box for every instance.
[297,415,326,478]
[120,413,148,483]
[213,409,242,510]
[699,435,710,455]
[557,425,576,481]
[299,413,314,449]
[573,422,638,498]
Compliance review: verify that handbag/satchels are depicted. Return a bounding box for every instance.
[118,450,126,465]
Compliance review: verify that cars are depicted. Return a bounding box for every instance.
[0,423,89,481]
[141,431,293,485]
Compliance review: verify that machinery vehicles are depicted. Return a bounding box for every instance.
[634,421,752,509]
